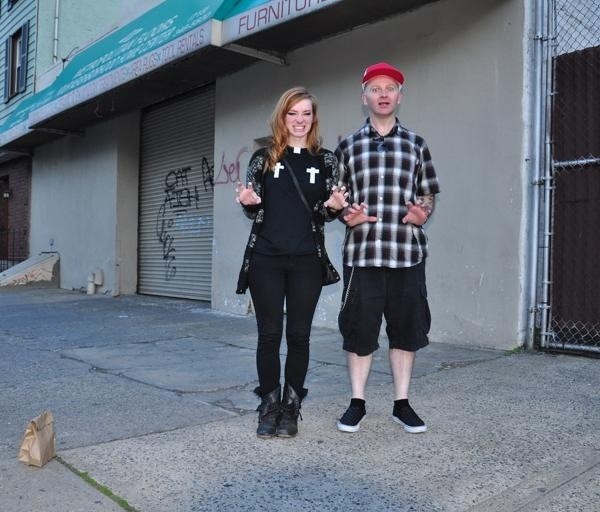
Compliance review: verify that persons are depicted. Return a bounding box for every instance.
[332,61,441,434]
[234,85,350,439]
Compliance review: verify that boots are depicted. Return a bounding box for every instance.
[253,382,308,438]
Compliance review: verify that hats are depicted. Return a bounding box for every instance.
[362,62,404,84]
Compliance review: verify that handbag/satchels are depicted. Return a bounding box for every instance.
[322,253,340,286]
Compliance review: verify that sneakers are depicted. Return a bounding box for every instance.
[392,404,427,433]
[337,403,366,432]
